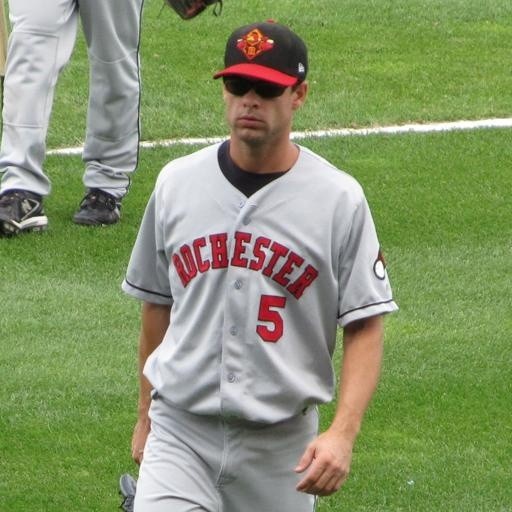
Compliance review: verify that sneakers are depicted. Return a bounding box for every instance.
[0,190,48,236]
[72,189,120,225]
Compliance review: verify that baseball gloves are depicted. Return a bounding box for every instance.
[166,0,222,20]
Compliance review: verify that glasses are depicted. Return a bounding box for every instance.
[225,79,288,97]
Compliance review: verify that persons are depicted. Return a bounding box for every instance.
[0,1,144,237]
[118,18,402,511]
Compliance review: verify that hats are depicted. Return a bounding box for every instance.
[213,18,307,86]
